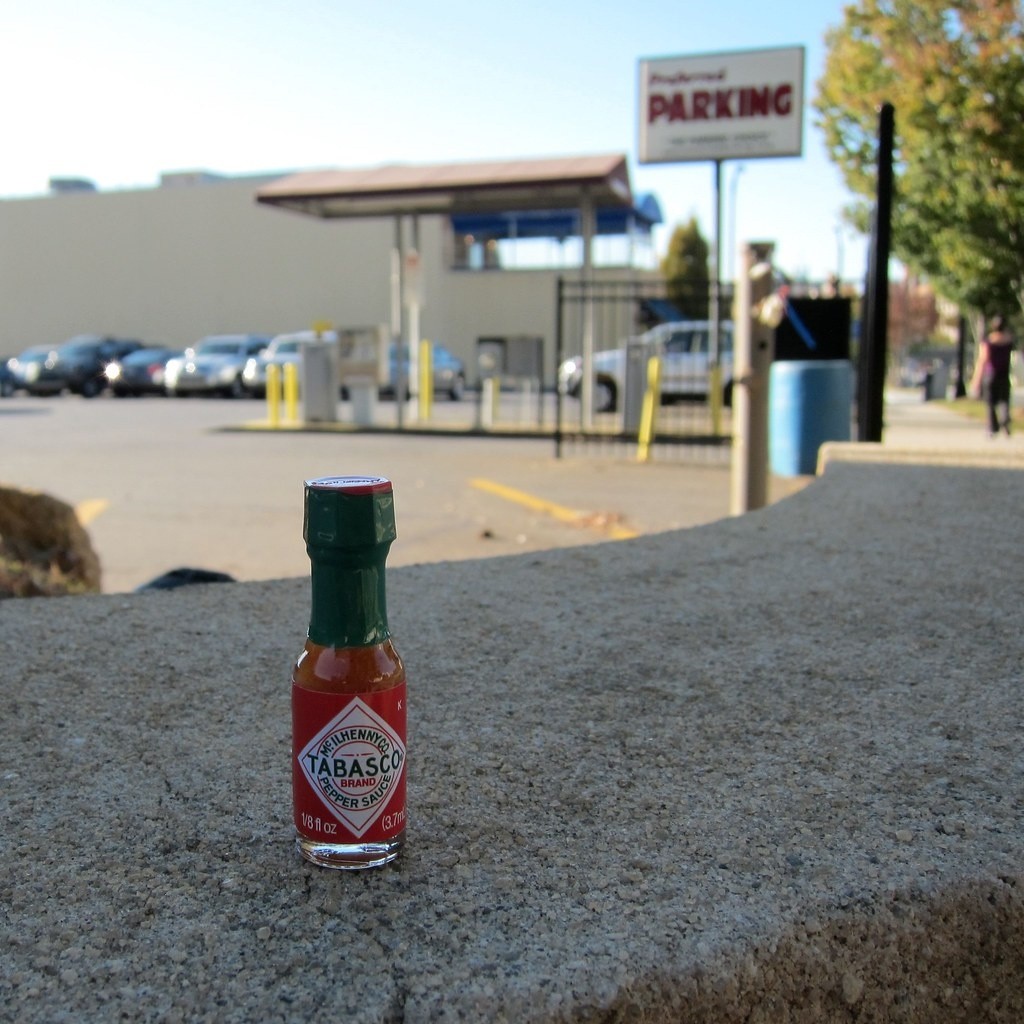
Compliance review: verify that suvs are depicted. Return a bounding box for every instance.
[557,317,733,412]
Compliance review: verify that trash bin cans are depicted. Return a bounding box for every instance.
[768,359,853,476]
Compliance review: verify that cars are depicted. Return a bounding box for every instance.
[3,330,464,401]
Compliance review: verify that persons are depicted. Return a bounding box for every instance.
[970,315,1015,435]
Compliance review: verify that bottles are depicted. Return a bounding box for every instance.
[291,477,408,870]
